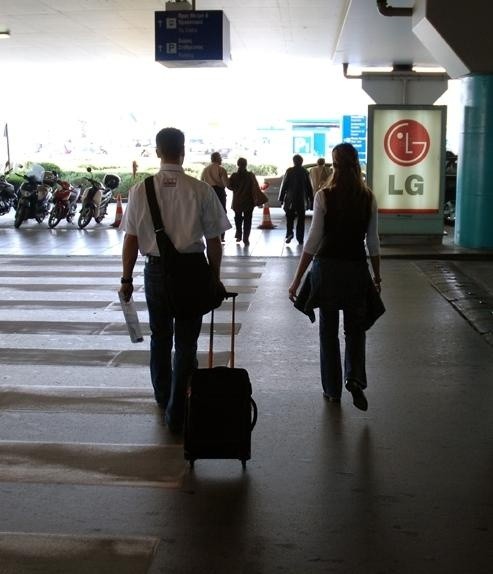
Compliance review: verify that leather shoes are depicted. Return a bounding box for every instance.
[158,400,177,434]
[322,381,368,412]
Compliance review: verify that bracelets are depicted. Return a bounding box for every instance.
[120,277,134,284]
[373,277,381,283]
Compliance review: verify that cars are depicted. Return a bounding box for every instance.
[258,163,367,217]
[443,151,458,227]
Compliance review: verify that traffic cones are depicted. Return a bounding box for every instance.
[257,198,278,228]
[109,194,123,227]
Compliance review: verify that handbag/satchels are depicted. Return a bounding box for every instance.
[162,252,222,318]
[252,183,268,205]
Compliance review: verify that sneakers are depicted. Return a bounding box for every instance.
[286,235,293,243]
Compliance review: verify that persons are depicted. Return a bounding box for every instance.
[276,155,316,245]
[308,157,333,206]
[119,127,232,435]
[288,142,385,411]
[227,157,264,245]
[200,152,231,242]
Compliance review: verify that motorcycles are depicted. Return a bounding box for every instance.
[0,160,120,229]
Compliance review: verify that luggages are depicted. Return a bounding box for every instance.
[184,292,258,467]
